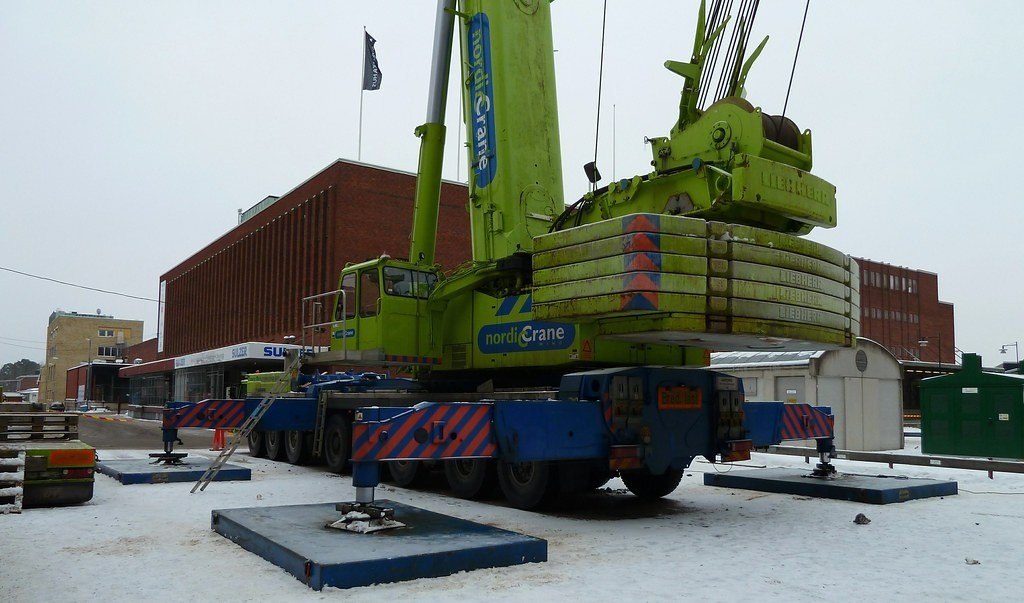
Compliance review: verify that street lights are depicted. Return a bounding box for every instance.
[47,361,55,402]
[85,337,91,400]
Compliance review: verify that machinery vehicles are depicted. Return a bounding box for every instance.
[323,1,862,397]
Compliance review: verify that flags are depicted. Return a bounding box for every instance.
[363,31,382,90]
